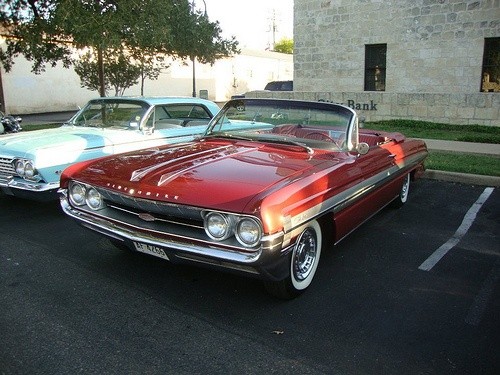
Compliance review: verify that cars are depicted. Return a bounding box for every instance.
[0,97,274,217]
[231,81,294,111]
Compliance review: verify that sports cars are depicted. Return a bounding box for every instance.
[57,98,430,295]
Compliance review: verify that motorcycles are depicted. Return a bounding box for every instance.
[0,112,22,135]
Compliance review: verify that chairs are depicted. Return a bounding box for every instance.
[153,123,182,130]
[338,132,385,147]
[157,119,186,126]
[298,127,332,141]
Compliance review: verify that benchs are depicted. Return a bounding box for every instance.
[308,125,392,140]
